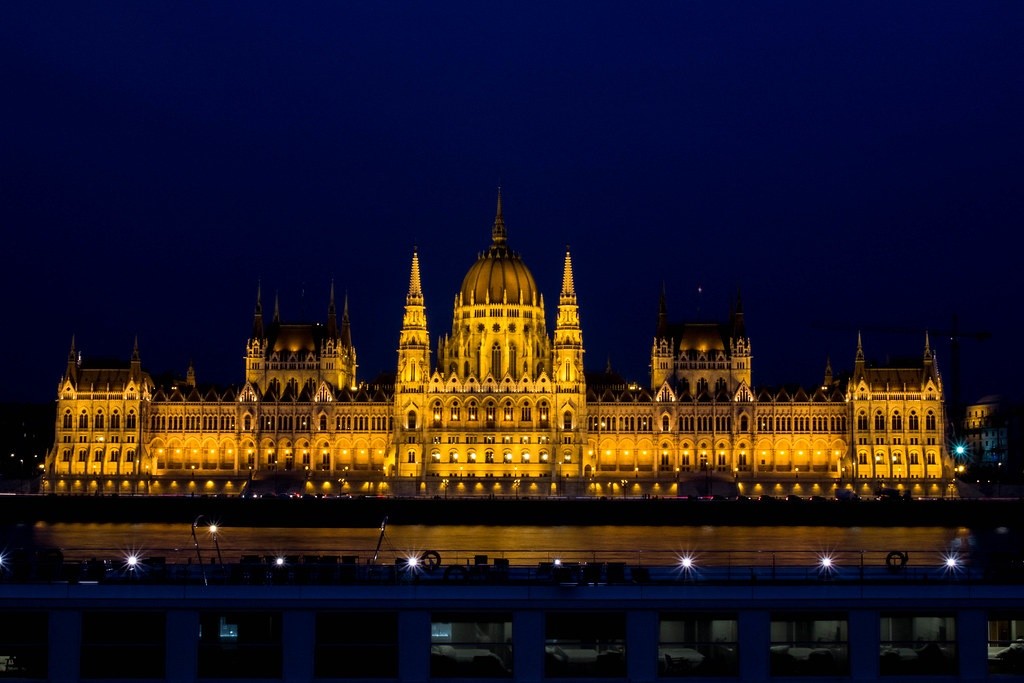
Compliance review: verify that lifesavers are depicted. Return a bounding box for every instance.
[887,550,905,566]
[421,551,441,569]
